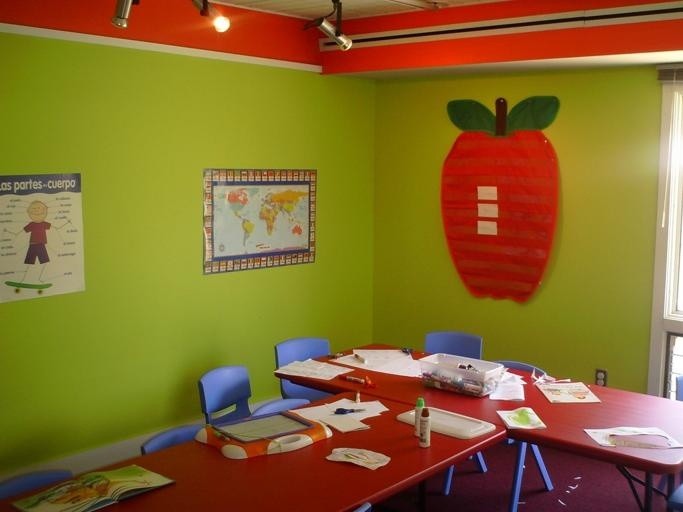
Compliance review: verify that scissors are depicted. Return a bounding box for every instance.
[334,407,365,414]
[402,347,413,356]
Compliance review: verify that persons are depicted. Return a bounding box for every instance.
[2,200,71,284]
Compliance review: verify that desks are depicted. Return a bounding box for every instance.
[0,380,510,510]
[271,343,683,508]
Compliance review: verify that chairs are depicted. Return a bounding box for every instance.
[422,332,487,495]
[198,365,254,428]
[248,398,310,421]
[0,467,72,498]
[481,356,552,512]
[134,423,214,454]
[270,336,335,401]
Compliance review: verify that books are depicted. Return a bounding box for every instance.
[13,464,175,511]
[488,372,600,429]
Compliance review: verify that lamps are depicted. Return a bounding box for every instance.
[105,0,140,27]
[304,1,354,55]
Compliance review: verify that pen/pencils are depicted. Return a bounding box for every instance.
[422,364,497,396]
[354,354,364,363]
[337,374,364,383]
[205,425,230,441]
